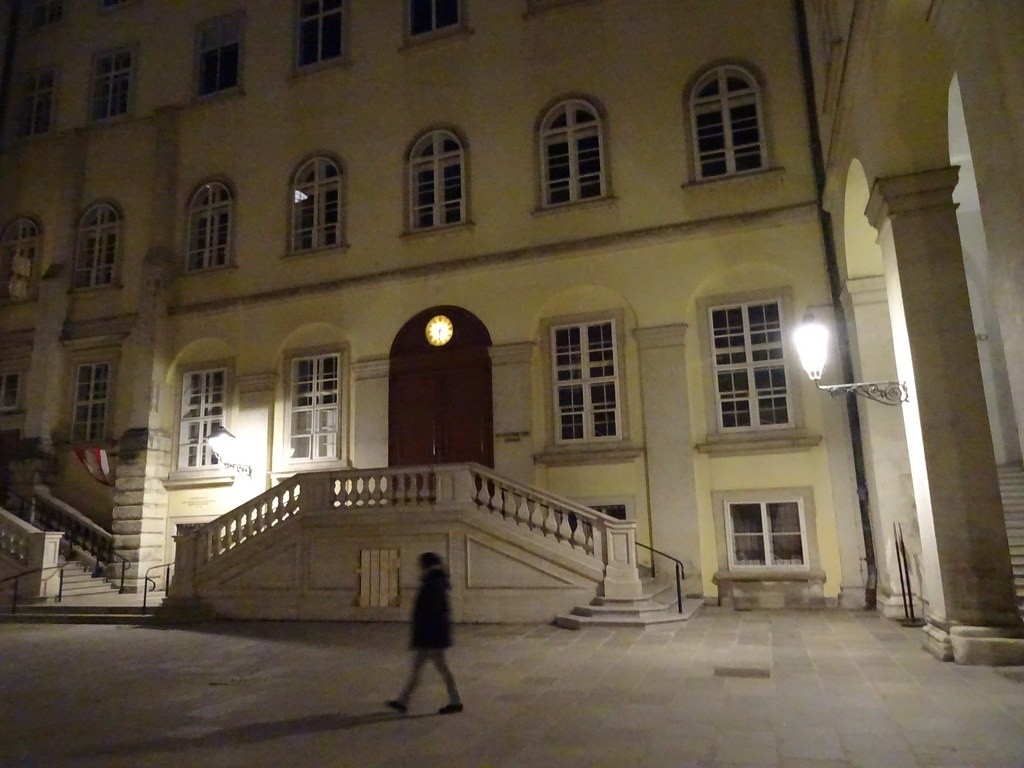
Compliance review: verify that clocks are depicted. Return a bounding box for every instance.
[425,315,453,346]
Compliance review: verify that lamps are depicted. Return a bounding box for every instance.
[793,303,910,408]
[207,426,254,477]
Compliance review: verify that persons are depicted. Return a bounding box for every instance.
[384,552,464,716]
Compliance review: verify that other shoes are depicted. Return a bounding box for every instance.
[440,705,464,713]
[385,699,409,713]
